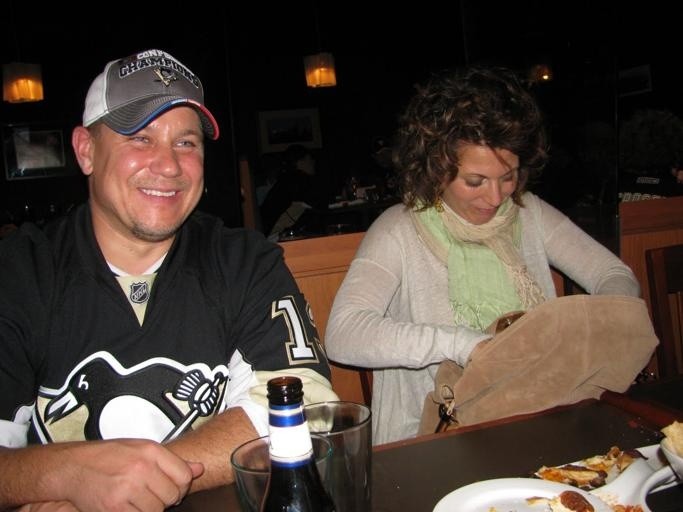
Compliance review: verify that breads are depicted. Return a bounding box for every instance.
[549,491,594,512]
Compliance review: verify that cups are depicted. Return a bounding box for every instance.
[300,401,374,512]
[228,430,334,512]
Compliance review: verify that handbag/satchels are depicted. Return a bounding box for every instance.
[416,294,660,438]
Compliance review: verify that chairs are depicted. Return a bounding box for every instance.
[265,230,374,406]
[617,196,682,383]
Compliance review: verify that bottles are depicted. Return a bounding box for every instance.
[257,373,338,512]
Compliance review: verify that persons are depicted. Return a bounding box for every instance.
[0,47,338,512]
[577,107,682,224]
[321,58,640,445]
[257,141,317,236]
[341,133,396,232]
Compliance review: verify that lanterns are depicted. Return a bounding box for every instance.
[522,63,557,85]
[0,60,48,106]
[299,51,339,90]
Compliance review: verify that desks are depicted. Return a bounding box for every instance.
[317,202,394,232]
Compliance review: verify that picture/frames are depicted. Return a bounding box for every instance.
[256,108,322,157]
[2,121,70,182]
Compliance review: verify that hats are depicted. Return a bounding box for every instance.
[83,49,220,140]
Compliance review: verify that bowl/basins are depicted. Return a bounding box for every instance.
[660,429,683,480]
[638,462,683,512]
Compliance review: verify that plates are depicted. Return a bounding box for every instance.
[532,438,683,496]
[588,457,667,512]
[431,476,617,512]
